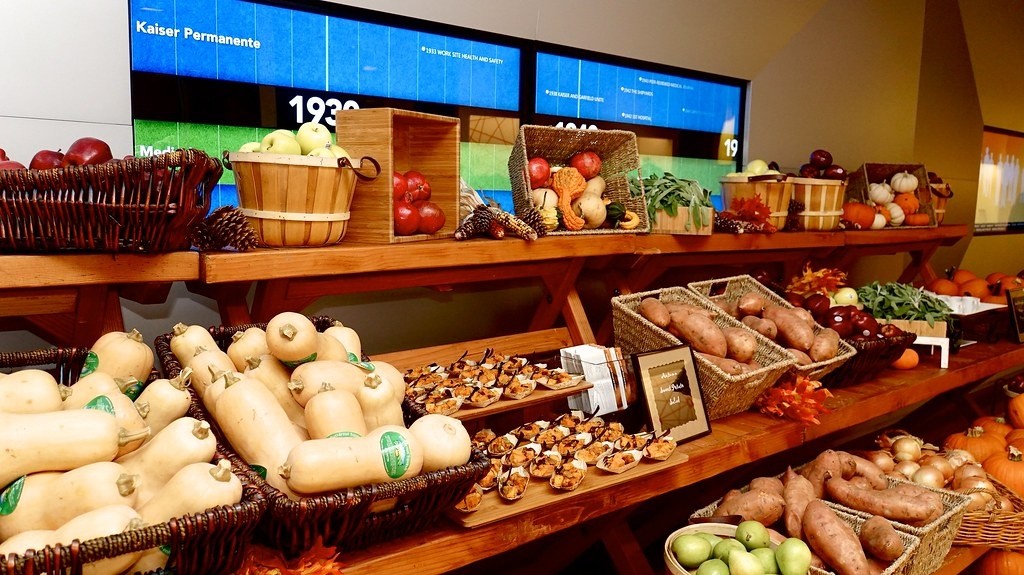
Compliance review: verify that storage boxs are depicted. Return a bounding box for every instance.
[820,332,917,389]
[0,148,224,254]
[651,207,715,236]
[155,314,491,561]
[689,483,919,575]
[718,173,792,230]
[876,318,947,338]
[508,124,651,237]
[221,148,382,248]
[776,465,972,574]
[791,177,843,230]
[611,285,799,420]
[929,182,953,225]
[0,346,271,575]
[850,161,939,230]
[688,274,857,382]
[931,452,1024,552]
[335,107,461,244]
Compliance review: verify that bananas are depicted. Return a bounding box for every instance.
[619,208,640,230]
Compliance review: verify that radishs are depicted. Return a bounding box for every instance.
[0,310,471,559]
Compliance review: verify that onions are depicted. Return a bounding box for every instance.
[866,438,1015,513]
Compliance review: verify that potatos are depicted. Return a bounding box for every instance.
[715,449,942,574]
[640,290,840,377]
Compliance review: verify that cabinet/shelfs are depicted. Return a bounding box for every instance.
[0,224,1024,573]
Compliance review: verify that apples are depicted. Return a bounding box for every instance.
[727,149,848,180]
[238,123,351,160]
[751,266,906,341]
[0,137,112,170]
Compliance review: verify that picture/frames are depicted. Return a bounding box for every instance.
[1006,288,1024,345]
[626,342,712,446]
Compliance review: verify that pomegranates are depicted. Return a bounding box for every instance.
[528,157,550,188]
[571,150,601,178]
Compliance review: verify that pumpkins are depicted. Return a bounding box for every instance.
[944,385,1024,499]
[869,169,929,228]
[925,265,1024,305]
[842,202,875,229]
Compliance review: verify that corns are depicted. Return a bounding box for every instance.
[454,204,538,241]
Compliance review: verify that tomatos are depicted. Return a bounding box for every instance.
[392,170,446,234]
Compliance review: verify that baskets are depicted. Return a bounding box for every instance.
[772,461,972,575]
[934,452,1024,553]
[509,124,651,235]
[688,483,921,575]
[687,274,857,381]
[610,286,797,420]
[154,314,491,558]
[818,333,917,388]
[1,346,270,575]
[1,148,224,252]
